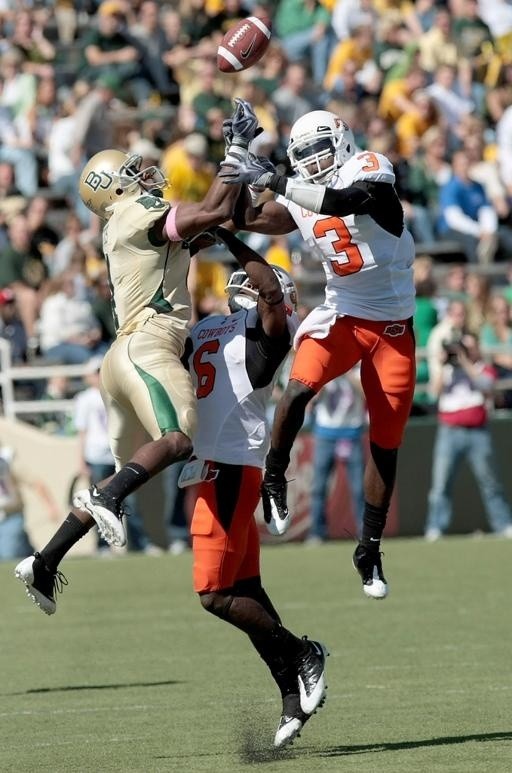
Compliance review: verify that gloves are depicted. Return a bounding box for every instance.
[181,97,277,252]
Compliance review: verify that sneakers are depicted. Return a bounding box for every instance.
[73,483,131,547]
[352,543,388,599]
[274,685,328,747]
[258,471,295,535]
[296,634,329,714]
[14,551,68,614]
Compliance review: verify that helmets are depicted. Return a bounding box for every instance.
[78,149,170,222]
[225,264,298,317]
[286,110,355,184]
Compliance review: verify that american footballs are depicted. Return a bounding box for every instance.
[217,15,273,72]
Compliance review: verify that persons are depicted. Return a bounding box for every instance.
[74,357,117,558]
[177,222,329,751]
[14,95,266,615]
[300,368,364,546]
[0,458,35,561]
[0,0,511,438]
[218,109,415,600]
[414,254,512,410]
[422,332,512,542]
